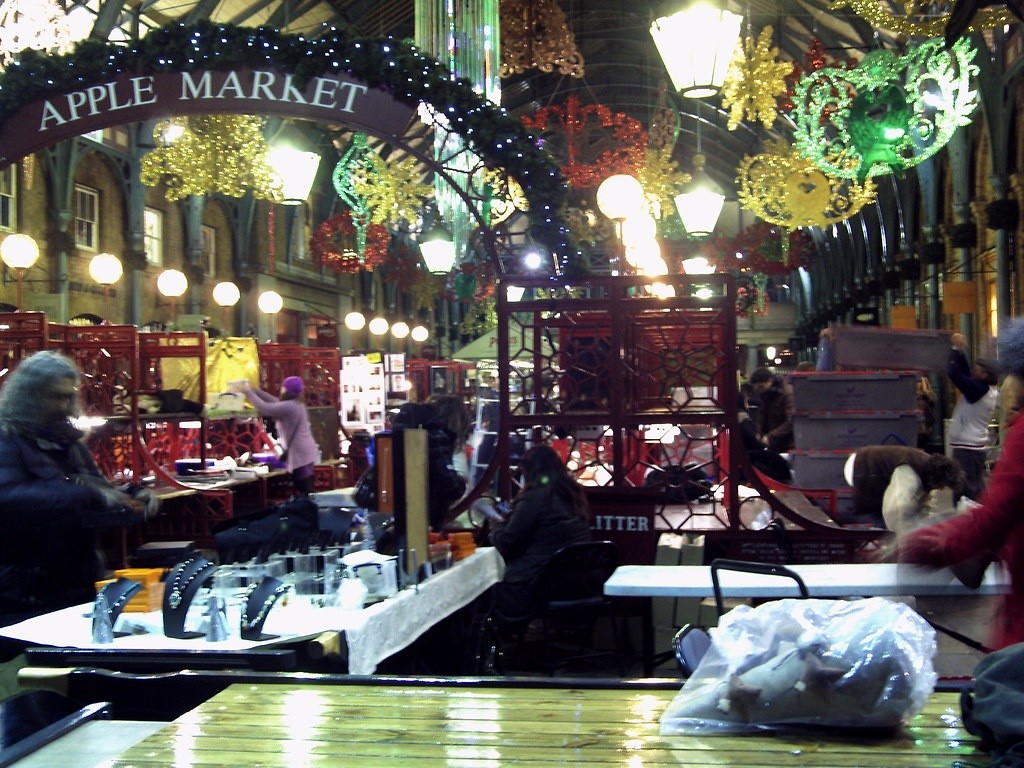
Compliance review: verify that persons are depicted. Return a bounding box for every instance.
[949,332,997,489]
[739,328,834,485]
[236,377,317,493]
[488,443,590,674]
[877,317,1024,648]
[0,352,158,662]
[1000,374,1024,445]
[845,445,966,550]
[918,376,945,444]
[339,414,370,481]
[361,374,557,534]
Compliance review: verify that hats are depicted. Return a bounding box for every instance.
[282,376,303,396]
[752,368,771,376]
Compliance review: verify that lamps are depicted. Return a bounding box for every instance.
[263,118,323,205]
[673,99,726,239]
[681,246,717,288]
[416,222,455,276]
[645,0,745,100]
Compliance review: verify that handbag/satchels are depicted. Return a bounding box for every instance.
[657,595,939,736]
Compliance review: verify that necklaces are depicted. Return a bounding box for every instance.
[89,558,292,629]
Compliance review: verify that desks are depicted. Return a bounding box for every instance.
[111,683,979,768]
[603,564,1014,678]
[0,547,507,676]
[213,510,393,565]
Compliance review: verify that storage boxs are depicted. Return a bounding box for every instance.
[784,369,924,411]
[809,492,862,516]
[789,451,850,489]
[786,408,923,451]
[836,326,952,371]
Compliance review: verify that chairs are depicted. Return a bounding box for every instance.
[671,623,711,679]
[509,539,624,677]
[710,559,810,617]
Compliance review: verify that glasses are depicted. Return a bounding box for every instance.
[751,375,769,383]
